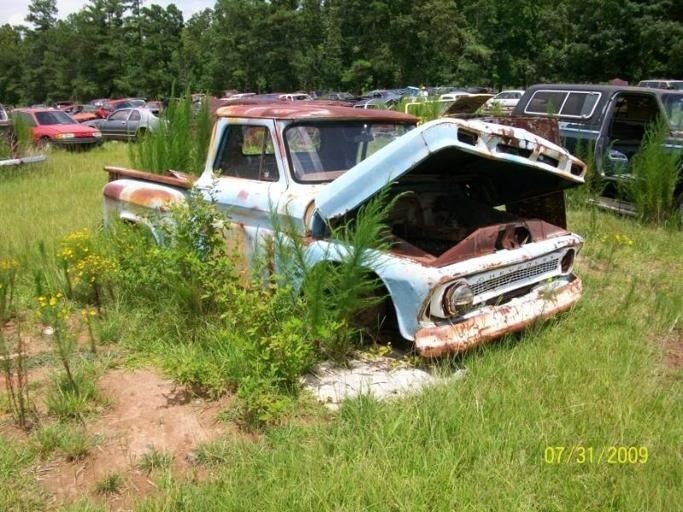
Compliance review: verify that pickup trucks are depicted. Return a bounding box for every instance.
[100,98,589,361]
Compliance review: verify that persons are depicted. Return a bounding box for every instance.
[417,86,428,96]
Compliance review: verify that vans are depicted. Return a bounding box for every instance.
[512,79,683,220]
